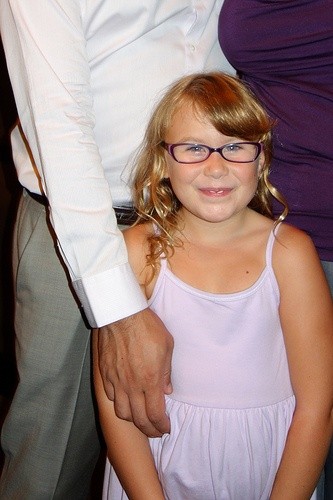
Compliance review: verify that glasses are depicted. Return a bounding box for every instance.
[160,140,264,164]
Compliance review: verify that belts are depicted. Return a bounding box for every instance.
[23,188,145,226]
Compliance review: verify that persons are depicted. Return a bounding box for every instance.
[0,0,236,500]
[92,72,333,500]
